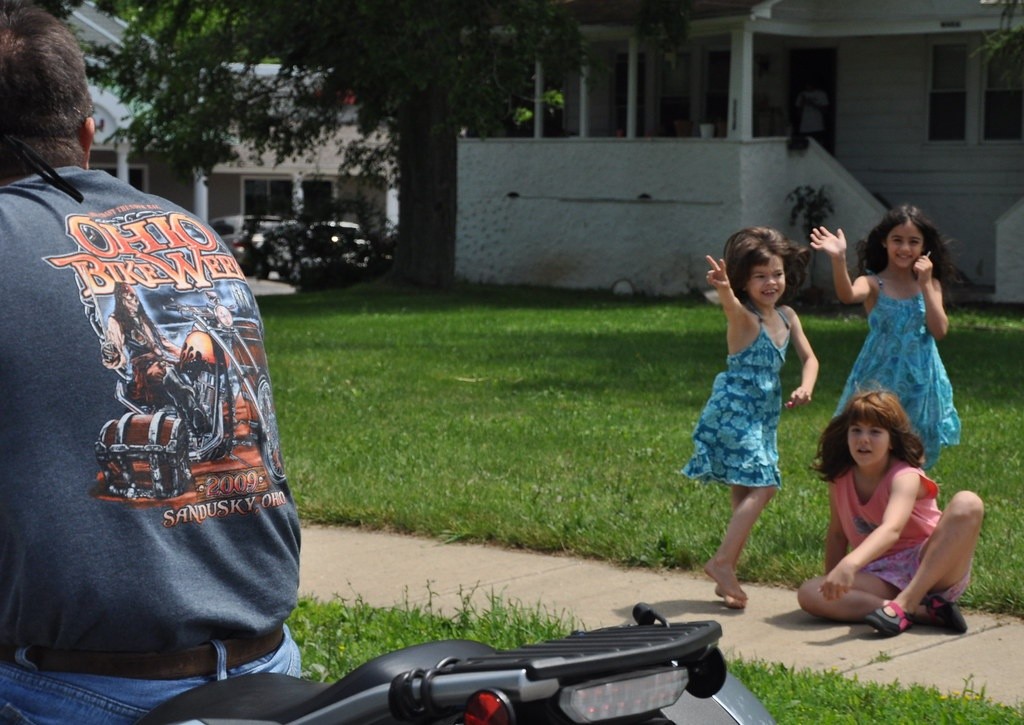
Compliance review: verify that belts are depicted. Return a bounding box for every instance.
[0,625,284,679]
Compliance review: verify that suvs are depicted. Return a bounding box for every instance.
[210,214,366,284]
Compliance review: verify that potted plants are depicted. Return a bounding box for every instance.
[785,185,834,307]
[698,111,721,138]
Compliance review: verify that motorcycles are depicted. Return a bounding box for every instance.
[126,603,773,725]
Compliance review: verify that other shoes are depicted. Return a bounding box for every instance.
[921,596,968,632]
[864,602,916,634]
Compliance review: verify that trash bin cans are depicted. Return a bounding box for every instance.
[778,240,811,308]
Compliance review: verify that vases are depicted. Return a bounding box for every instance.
[715,120,727,138]
[674,119,695,137]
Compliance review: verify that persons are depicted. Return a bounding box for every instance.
[681,226,820,610]
[797,390,984,635]
[0,0,303,725]
[809,203,971,472]
